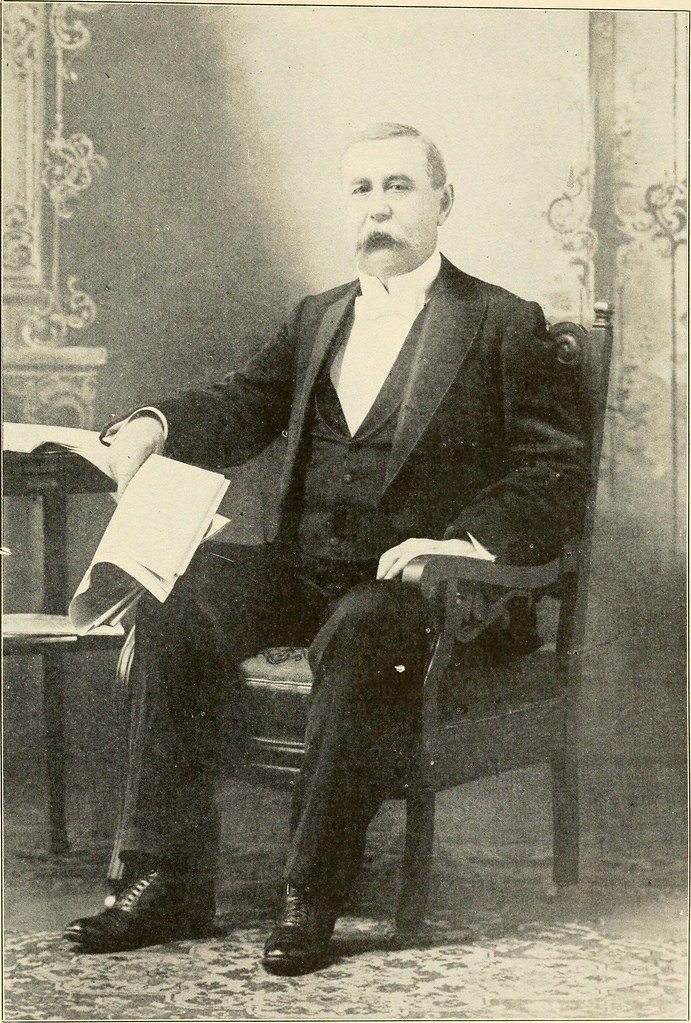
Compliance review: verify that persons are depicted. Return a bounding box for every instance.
[61,122,590,973]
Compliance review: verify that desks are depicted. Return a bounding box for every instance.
[4,444,126,856]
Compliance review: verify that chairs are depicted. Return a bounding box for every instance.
[103,300,615,948]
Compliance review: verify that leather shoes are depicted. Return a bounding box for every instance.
[264,887,338,974]
[62,864,215,949]
